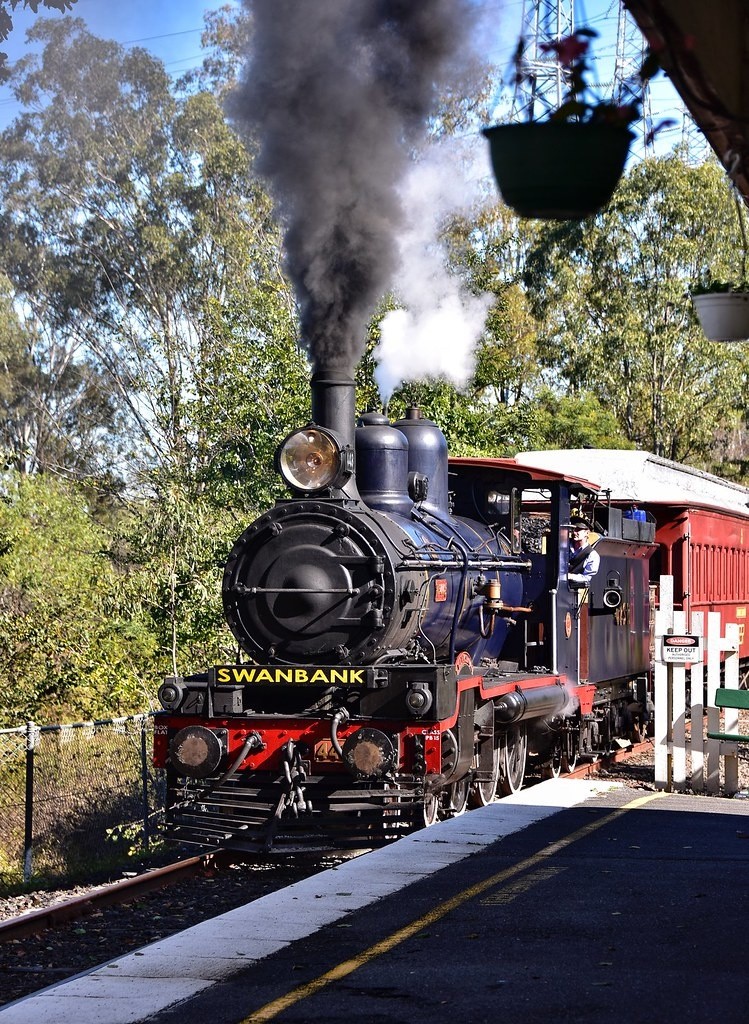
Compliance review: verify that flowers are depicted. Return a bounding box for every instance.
[506,22,698,145]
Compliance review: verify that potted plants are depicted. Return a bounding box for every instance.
[683,271,749,342]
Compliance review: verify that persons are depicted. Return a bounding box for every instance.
[568,516,600,583]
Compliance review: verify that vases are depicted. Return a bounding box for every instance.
[480,123,637,220]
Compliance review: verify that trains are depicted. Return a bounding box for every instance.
[146,375,749,842]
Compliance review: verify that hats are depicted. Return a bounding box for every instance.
[570,515,595,531]
